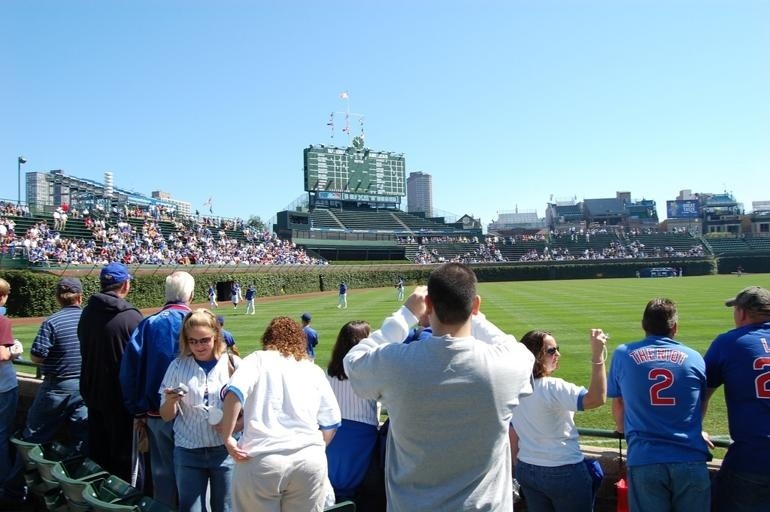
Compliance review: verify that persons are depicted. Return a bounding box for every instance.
[220,317,343,512]
[0,266,246,511]
[679,267,682,277]
[407,220,703,264]
[673,268,677,276]
[403,284,432,343]
[325,320,381,500]
[703,286,770,512]
[1,200,310,267]
[337,281,347,309]
[394,276,405,301]
[245,284,256,315]
[509,328,608,511]
[606,299,713,511]
[737,265,742,277]
[300,313,318,363]
[636,271,640,280]
[343,263,536,511]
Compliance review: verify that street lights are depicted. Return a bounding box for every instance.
[16,154,28,203]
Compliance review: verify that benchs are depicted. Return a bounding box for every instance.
[0,214,282,257]
[308,207,769,264]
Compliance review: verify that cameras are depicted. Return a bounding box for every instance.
[173,383,189,396]
[599,330,610,341]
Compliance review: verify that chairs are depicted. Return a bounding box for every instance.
[322,500,356,512]
[7,422,176,512]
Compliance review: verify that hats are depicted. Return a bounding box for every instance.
[725,286,770,307]
[216,315,224,322]
[301,312,311,320]
[100,262,134,282]
[58,278,81,293]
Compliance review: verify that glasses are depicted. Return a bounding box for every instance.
[548,346,559,354]
[187,337,211,344]
